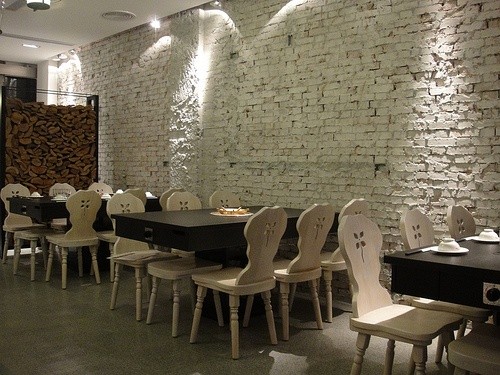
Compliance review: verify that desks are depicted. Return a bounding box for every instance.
[3,195,163,267]
[383,234,500,313]
[110,206,339,321]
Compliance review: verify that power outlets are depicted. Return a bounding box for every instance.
[482,282,500,307]
[145,227,153,243]
[22,205,26,216]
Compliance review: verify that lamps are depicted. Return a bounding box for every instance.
[27,0,50,11]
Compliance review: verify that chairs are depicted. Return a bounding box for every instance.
[0,181,500,375]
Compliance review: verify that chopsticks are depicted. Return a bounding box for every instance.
[405,250,422,256]
[456,239,465,242]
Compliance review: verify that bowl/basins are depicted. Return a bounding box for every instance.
[145,192,152,197]
[438,238,460,252]
[31,192,40,196]
[102,193,110,197]
[116,190,123,193]
[478,229,498,240]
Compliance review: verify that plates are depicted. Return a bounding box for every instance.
[26,196,44,197]
[219,209,247,214]
[100,197,112,199]
[471,236,500,242]
[51,197,70,202]
[430,247,469,253]
[146,197,157,199]
